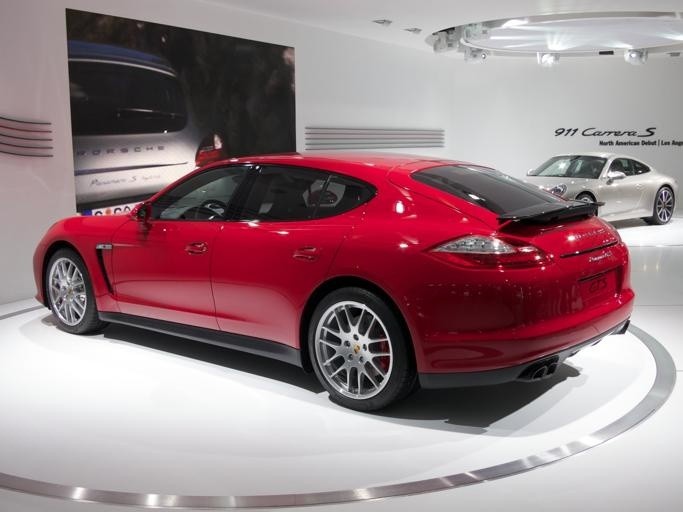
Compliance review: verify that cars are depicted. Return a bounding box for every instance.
[30,153,634,403]
[64,33,237,216]
[518,152,676,230]
